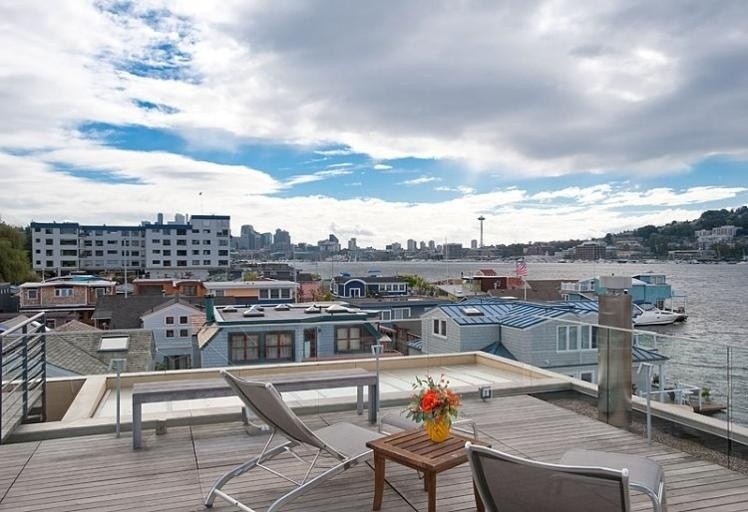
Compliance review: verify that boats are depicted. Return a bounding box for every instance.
[633,299,687,327]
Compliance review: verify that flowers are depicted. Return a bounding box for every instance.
[400,373,463,418]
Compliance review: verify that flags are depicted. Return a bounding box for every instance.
[516,261,528,277]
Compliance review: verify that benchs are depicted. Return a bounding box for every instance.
[132,368,379,449]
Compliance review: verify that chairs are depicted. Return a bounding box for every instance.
[465,438,667,512]
[203,365,392,512]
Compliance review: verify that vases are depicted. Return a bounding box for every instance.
[423,419,452,441]
